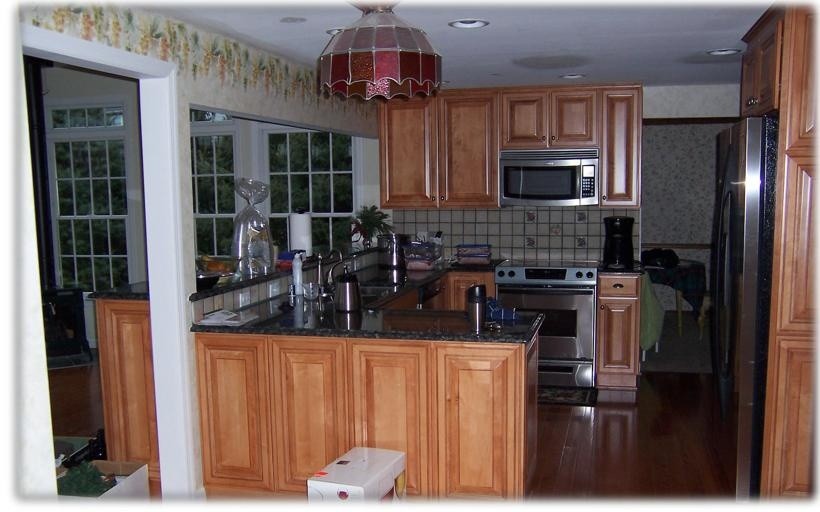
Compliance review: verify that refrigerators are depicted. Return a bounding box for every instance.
[710,116,766,498]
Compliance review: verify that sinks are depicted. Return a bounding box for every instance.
[359,286,398,308]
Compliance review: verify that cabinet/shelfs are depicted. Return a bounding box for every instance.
[501,85,598,148]
[597,275,642,390]
[449,271,495,311]
[377,88,498,209]
[598,84,643,209]
[739,19,783,118]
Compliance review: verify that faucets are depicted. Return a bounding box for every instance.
[326,254,357,284]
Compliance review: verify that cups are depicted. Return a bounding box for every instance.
[302,281,318,301]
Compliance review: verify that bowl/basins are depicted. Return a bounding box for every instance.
[196,257,241,290]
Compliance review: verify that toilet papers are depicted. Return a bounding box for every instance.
[286,212,313,258]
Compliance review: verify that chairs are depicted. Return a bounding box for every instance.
[641,272,664,361]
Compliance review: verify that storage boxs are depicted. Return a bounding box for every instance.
[57,460,151,499]
[307,447,406,503]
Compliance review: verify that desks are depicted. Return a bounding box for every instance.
[645,259,707,338]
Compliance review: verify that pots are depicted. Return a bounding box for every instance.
[376,233,411,269]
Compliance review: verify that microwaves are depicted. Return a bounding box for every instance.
[499,158,598,206]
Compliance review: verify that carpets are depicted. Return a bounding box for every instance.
[537,385,598,407]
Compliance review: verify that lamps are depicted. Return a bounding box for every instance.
[320,4,442,101]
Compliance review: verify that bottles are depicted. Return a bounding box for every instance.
[289,208,312,258]
[467,283,487,335]
[292,253,303,295]
[334,264,361,313]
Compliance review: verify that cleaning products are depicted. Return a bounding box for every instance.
[291,251,304,295]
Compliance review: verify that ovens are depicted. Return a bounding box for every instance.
[497,267,597,387]
